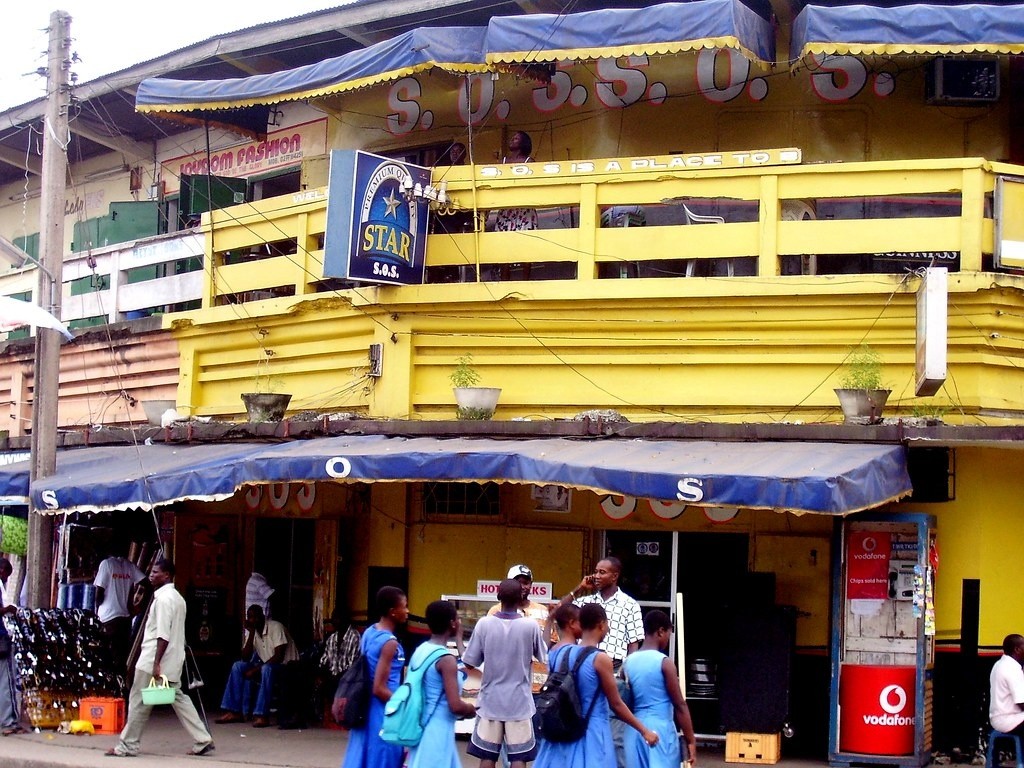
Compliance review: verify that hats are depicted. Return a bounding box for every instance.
[507,564,533,581]
[499,579,522,600]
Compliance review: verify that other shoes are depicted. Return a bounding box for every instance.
[186,741,215,755]
[251,715,270,727]
[214,712,244,724]
[104,748,136,757]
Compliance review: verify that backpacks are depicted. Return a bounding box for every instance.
[330,650,369,729]
[531,645,607,743]
[378,648,467,748]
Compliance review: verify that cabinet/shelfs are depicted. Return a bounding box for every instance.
[440,592,562,741]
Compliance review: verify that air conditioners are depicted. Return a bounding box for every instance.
[925,53,1001,107]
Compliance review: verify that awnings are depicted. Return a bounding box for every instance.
[0,434,915,519]
[485,0,776,85]
[788,4,1024,78]
[134,27,513,113]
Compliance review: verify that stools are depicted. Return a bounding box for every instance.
[986,730,1024,768]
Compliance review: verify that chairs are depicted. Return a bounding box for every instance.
[755,199,818,276]
[682,203,735,278]
[236,251,291,305]
[601,204,646,279]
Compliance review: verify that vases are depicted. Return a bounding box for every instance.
[141,400,177,426]
[240,392,293,423]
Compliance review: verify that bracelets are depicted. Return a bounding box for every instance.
[569,591,576,600]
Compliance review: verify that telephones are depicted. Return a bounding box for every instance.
[888,559,918,601]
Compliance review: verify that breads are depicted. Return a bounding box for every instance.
[456,608,490,628]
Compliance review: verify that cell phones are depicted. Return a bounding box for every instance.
[591,577,595,584]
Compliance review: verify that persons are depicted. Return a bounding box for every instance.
[402,601,477,768]
[989,634,1024,735]
[0,558,29,734]
[104,560,215,757]
[494,131,538,280]
[487,556,697,768]
[214,605,301,727]
[242,563,276,650]
[314,609,361,723]
[439,142,467,282]
[456,579,563,768]
[343,587,408,768]
[93,549,152,672]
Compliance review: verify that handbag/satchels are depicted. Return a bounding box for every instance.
[143,674,177,706]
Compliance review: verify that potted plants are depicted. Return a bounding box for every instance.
[831,341,893,425]
[452,353,504,420]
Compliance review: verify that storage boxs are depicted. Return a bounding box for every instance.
[78,697,126,735]
[725,731,782,765]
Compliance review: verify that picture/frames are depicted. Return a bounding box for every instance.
[529,482,572,514]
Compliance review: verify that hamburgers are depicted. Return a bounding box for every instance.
[459,668,483,699]
